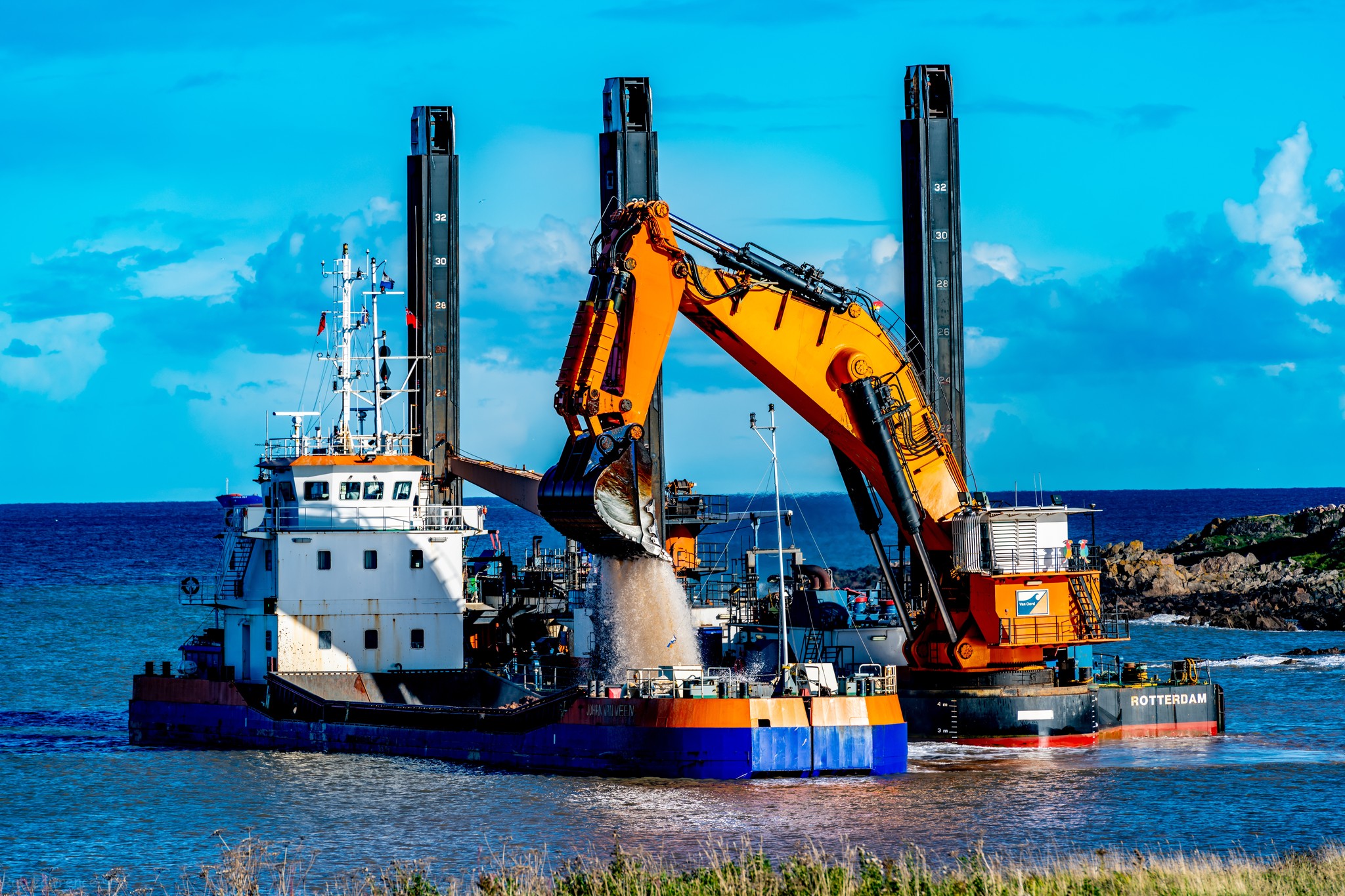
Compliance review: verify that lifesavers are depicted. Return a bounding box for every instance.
[182,576,200,596]
[225,509,234,527]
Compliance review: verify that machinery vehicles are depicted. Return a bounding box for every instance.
[532,192,1133,690]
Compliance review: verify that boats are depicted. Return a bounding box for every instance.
[127,241,912,785]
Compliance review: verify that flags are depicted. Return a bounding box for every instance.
[360,303,371,326]
[317,310,329,336]
[380,272,395,290]
[404,305,422,330]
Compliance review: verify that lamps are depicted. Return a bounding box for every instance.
[333,425,338,435]
[382,330,385,336]
[356,369,361,376]
[415,379,420,389]
[1050,494,1063,506]
[1024,579,1043,585]
[333,381,337,390]
[356,320,361,327]
[719,614,736,619]
[806,636,815,640]
[292,537,448,543]
[730,585,741,593]
[957,492,972,507]
[870,635,887,640]
[383,375,387,381]
[339,366,343,375]
[973,491,990,505]
[783,575,794,584]
[365,454,376,462]
[767,575,780,583]
[755,634,766,639]
[343,243,385,292]
